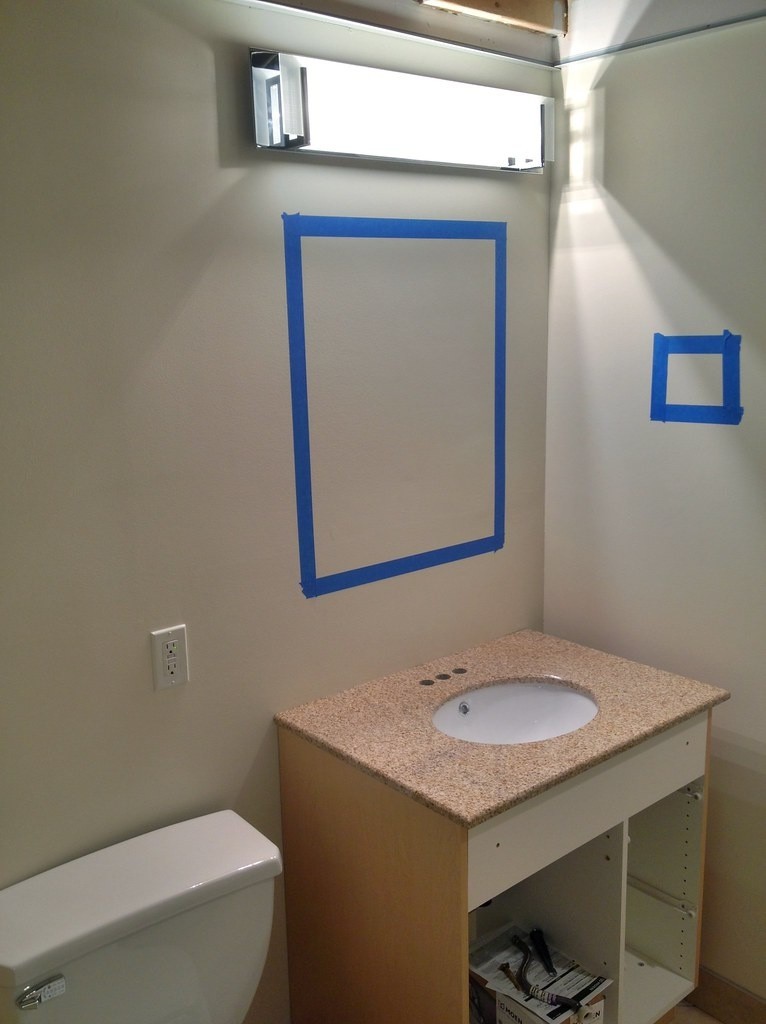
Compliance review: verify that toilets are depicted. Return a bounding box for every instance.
[0,808,285,1023]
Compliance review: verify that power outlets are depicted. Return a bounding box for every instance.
[150,623,190,692]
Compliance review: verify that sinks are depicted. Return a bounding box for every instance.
[430,676,601,746]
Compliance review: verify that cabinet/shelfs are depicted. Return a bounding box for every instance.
[272,708,714,1024]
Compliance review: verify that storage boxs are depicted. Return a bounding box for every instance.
[468,928,606,1022]
